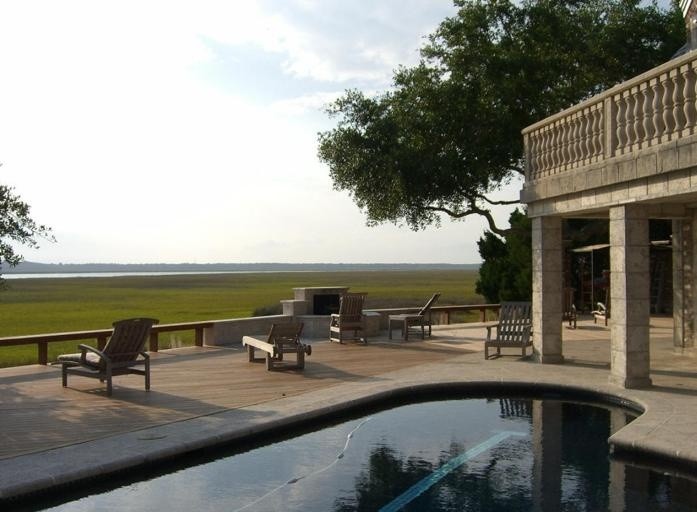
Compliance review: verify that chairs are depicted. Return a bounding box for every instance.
[57,316,159,395]
[242,322,311,371]
[590,287,610,326]
[484,301,534,360]
[562,287,576,328]
[329,291,367,345]
[401,291,440,338]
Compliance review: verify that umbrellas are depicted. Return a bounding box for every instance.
[570,244,610,310]
